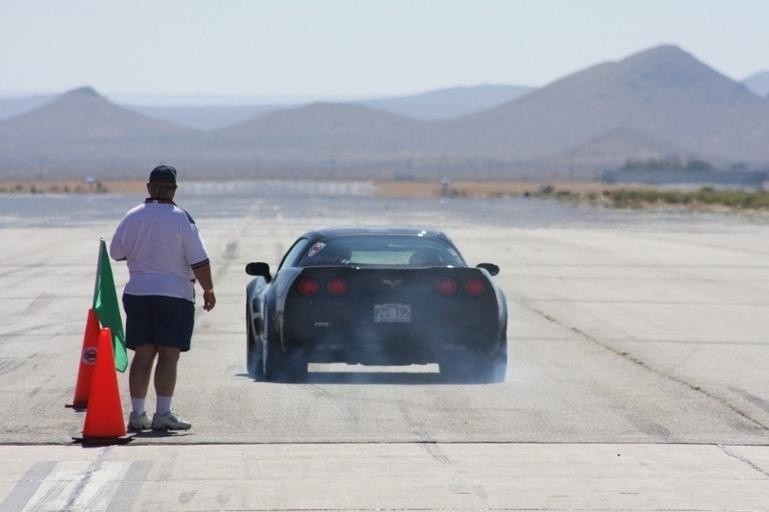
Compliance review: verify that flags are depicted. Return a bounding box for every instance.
[94,239,128,373]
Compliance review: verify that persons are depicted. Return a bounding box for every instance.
[110,165,216,431]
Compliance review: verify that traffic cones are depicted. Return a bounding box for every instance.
[65,309,137,444]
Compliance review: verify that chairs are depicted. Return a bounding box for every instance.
[409,250,441,266]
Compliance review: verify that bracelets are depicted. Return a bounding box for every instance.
[206,289,214,294]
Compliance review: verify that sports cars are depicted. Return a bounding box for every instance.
[246,226,507,384]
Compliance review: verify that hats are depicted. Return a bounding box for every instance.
[150,164,177,185]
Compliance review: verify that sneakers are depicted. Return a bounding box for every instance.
[152,411,191,430]
[127,411,152,431]
